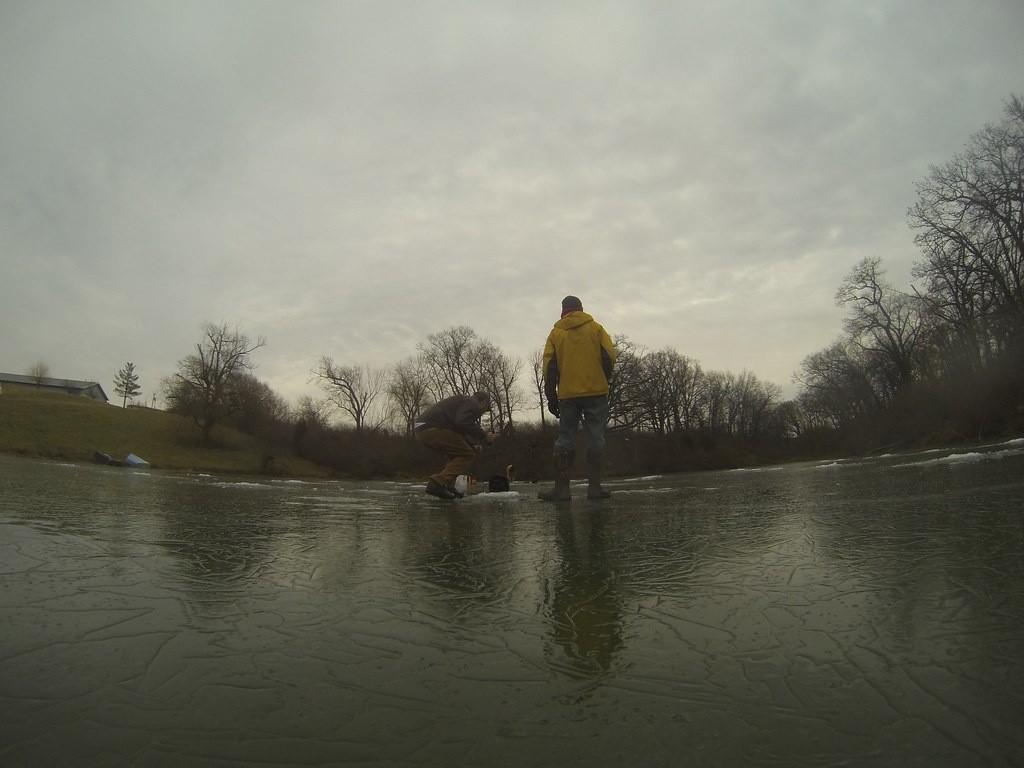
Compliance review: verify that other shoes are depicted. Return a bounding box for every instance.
[538,476,572,500]
[448,487,464,498]
[426,478,456,499]
[587,480,611,498]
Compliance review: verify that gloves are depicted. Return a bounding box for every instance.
[548,393,560,418]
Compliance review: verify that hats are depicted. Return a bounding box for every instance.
[474,392,491,411]
[562,296,582,310]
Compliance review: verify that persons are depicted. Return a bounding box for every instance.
[414,391,496,498]
[537,296,615,501]
